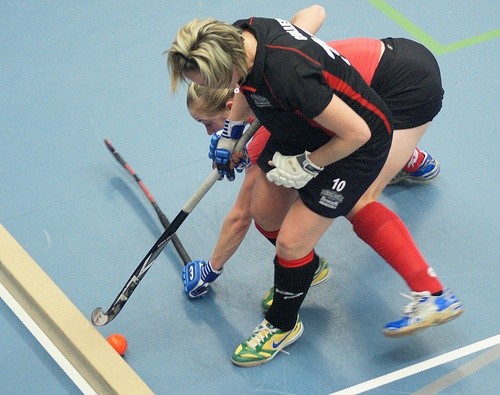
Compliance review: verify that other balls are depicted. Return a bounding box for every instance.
[106,333,128,356]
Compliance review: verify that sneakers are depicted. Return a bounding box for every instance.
[231,311,304,366]
[388,149,440,187]
[381,285,465,337]
[261,255,332,310]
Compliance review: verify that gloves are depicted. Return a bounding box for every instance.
[208,119,252,181]
[182,259,223,298]
[265,151,323,189]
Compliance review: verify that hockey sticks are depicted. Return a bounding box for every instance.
[104,138,192,266]
[92,118,260,325]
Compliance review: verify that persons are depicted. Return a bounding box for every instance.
[163,16,394,368]
[178,3,467,339]
